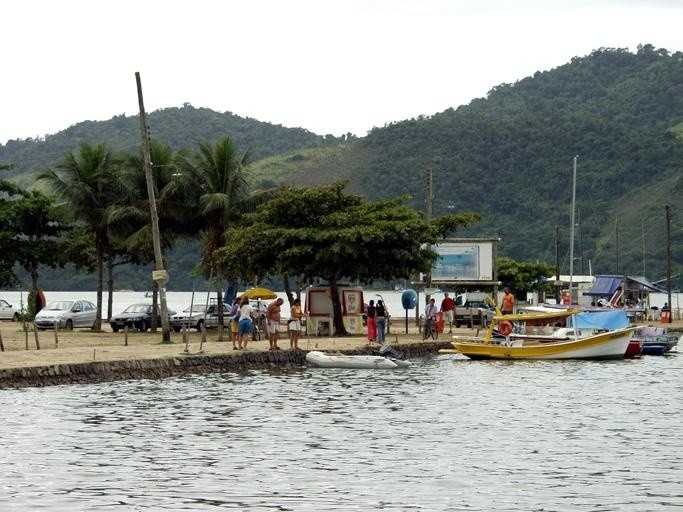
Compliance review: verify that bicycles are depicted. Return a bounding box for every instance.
[422,314,438,340]
[250,314,264,341]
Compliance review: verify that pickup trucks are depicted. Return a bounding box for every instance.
[453,299,494,328]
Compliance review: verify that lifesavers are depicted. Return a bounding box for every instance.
[498,321,512,334]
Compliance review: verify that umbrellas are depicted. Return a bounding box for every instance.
[241,287,276,308]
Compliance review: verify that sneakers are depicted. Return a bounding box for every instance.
[233,347,246,350]
[289,347,300,350]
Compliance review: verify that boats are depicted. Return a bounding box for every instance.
[305,350,412,369]
[449,307,679,360]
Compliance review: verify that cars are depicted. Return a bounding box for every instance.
[109,303,181,332]
[168,302,231,331]
[0,299,29,322]
[33,300,96,330]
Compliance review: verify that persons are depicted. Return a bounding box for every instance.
[374,300,387,344]
[663,303,667,309]
[230,298,243,350]
[265,297,284,350]
[289,298,305,350]
[501,286,514,315]
[238,295,254,351]
[442,292,455,333]
[424,298,437,340]
[365,299,378,342]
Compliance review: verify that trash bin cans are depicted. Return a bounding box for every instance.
[434,312,444,333]
[661,306,671,323]
